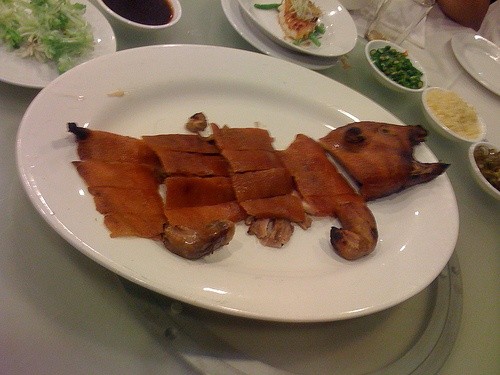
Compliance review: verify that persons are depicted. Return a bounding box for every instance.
[437,0,500,50]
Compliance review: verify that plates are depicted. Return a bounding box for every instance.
[469,142,500,199]
[96,0,181,30]
[364,40,429,93]
[451,32,500,96]
[421,87,486,144]
[0,0,118,88]
[15,44,460,322]
[221,0,357,70]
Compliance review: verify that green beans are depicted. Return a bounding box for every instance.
[309,23,325,47]
[254,3,281,9]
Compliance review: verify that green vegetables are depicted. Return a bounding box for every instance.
[371,44,423,90]
[0,0,96,74]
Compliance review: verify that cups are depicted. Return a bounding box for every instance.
[365,0,437,45]
